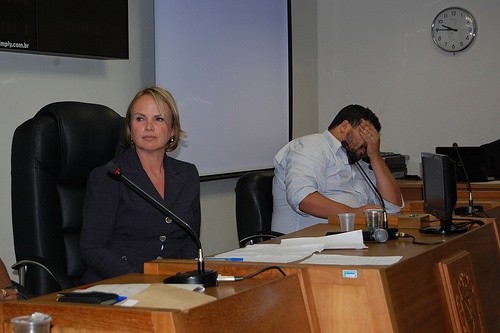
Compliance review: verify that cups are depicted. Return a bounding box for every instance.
[12,315,52,333]
[365,209,384,230]
[338,212,355,232]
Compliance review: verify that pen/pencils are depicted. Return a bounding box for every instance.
[203,256,245,262]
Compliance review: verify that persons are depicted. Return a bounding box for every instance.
[271,104,405,239]
[81,86,201,286]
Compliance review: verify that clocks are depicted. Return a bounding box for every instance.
[430,6,478,55]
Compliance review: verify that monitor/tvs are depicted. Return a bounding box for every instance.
[435,147,486,181]
[418,152,468,235]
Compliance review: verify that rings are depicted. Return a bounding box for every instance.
[370,136,372,139]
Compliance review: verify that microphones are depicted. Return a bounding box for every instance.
[341,140,399,240]
[452,143,483,216]
[326,228,389,243]
[106,162,218,286]
[386,228,410,239]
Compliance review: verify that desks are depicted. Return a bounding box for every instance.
[397,180,500,202]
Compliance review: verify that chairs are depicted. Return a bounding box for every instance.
[235,172,285,248]
[11,101,134,296]
